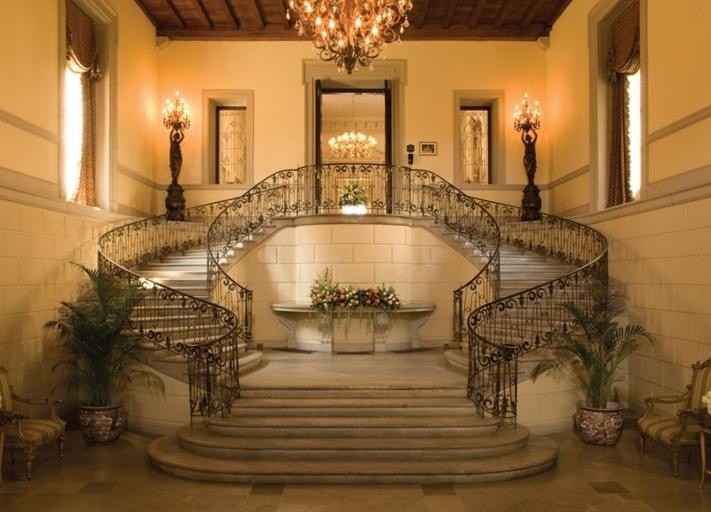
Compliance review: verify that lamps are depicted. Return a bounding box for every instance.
[162,91,191,130]
[328,132,378,160]
[513,93,541,132]
[285,0,413,75]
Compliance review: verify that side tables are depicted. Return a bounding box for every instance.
[698,427,711,489]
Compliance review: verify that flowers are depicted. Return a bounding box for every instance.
[702,391,711,415]
[309,266,400,310]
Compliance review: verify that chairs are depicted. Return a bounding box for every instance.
[0,365,66,480]
[638,356,711,477]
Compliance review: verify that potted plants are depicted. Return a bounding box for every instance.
[43,262,165,443]
[531,289,654,447]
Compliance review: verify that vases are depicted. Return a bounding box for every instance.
[702,415,711,429]
